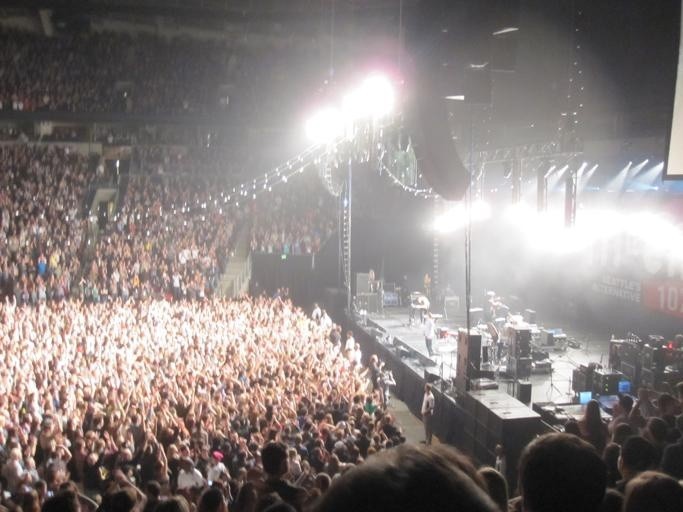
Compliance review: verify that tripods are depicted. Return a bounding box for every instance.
[546,363,562,398]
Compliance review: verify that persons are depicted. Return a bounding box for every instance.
[609,396,634,436]
[631,379,683,442]
[496,318,518,364]
[660,444,683,481]
[310,442,508,512]
[1,123,252,301]
[156,496,191,512]
[260,442,310,510]
[577,398,611,456]
[618,437,656,480]
[613,422,632,445]
[143,480,167,512]
[40,491,83,511]
[623,471,683,511]
[519,432,607,512]
[0,24,338,124]
[0,442,260,496]
[197,488,229,512]
[483,290,500,323]
[0,286,403,442]
[58,481,99,512]
[288,448,302,481]
[422,311,435,357]
[96,488,139,512]
[250,159,333,256]
[421,383,435,448]
[596,398,620,419]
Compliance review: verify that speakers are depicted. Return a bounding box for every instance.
[406,94,471,202]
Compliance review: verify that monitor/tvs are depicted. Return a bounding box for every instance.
[578,391,592,404]
[617,380,631,393]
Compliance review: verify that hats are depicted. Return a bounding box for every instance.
[212,451,223,460]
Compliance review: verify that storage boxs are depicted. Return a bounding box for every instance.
[643,345,659,368]
[592,367,623,394]
[609,338,642,363]
[621,362,637,381]
[510,357,532,378]
[572,369,592,393]
[641,368,656,384]
[541,329,553,345]
[518,380,531,402]
[524,309,536,323]
